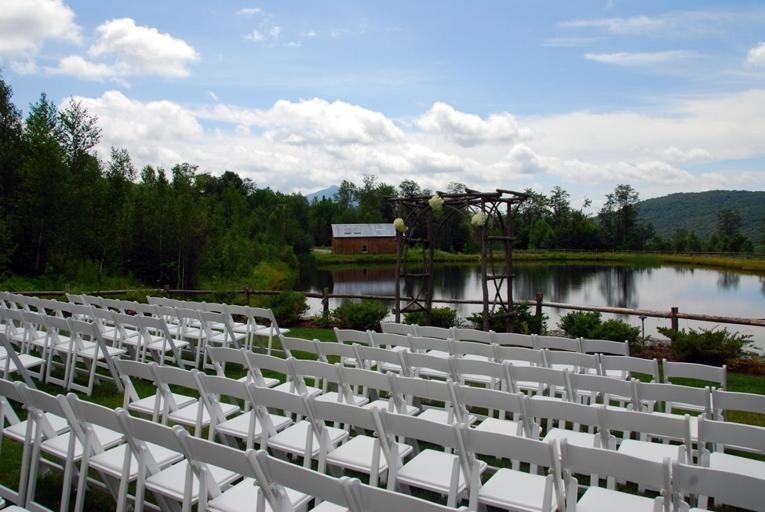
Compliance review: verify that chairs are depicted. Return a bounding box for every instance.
[0,290,765,512]
